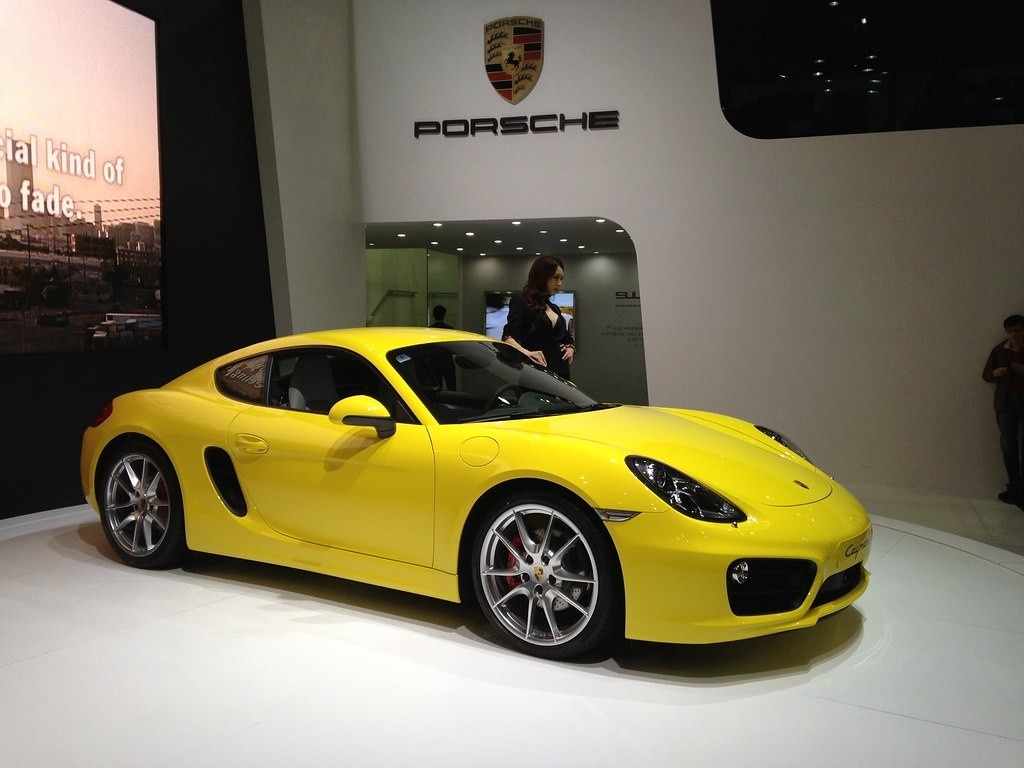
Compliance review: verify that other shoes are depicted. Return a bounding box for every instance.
[998,484,1024,505]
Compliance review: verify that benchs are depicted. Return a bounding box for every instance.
[375,355,421,425]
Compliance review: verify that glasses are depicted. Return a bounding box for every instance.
[1005,328,1023,333]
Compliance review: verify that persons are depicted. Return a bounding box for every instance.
[981,314,1024,511]
[427,305,458,391]
[501,255,577,389]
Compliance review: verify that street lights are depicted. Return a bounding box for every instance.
[22,224,32,281]
[63,232,74,305]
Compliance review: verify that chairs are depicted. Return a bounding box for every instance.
[289,352,340,415]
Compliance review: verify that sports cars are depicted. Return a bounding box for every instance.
[80,325,875,663]
